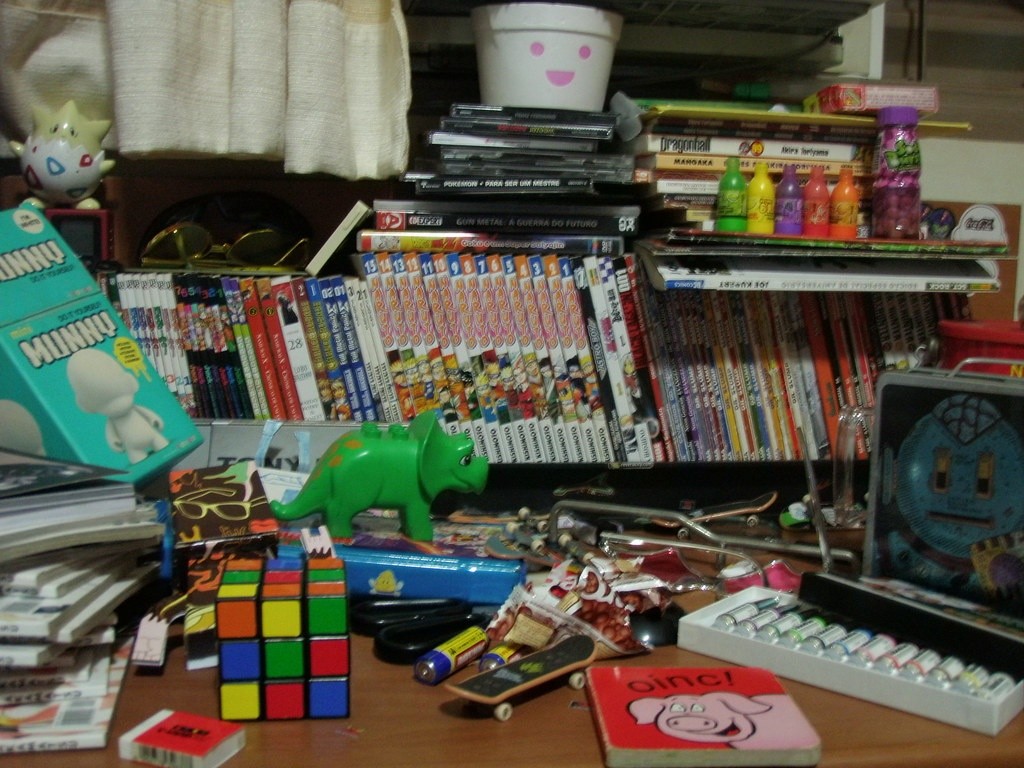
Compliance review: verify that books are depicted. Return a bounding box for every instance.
[0,102,972,768]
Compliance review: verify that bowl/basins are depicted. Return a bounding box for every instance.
[469,2,624,111]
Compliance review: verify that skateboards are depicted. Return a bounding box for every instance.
[428,488,576,522]
[601,530,769,588]
[650,490,779,540]
[441,634,598,722]
[485,521,596,572]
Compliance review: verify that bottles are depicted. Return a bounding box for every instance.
[774,165,804,238]
[803,166,831,240]
[714,157,748,233]
[745,161,775,235]
[829,168,859,240]
[870,105,922,241]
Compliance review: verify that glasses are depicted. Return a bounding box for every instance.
[139,222,308,268]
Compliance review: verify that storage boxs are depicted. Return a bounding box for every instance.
[0,202,206,491]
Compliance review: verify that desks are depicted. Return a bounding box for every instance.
[0,520,1024,768]
